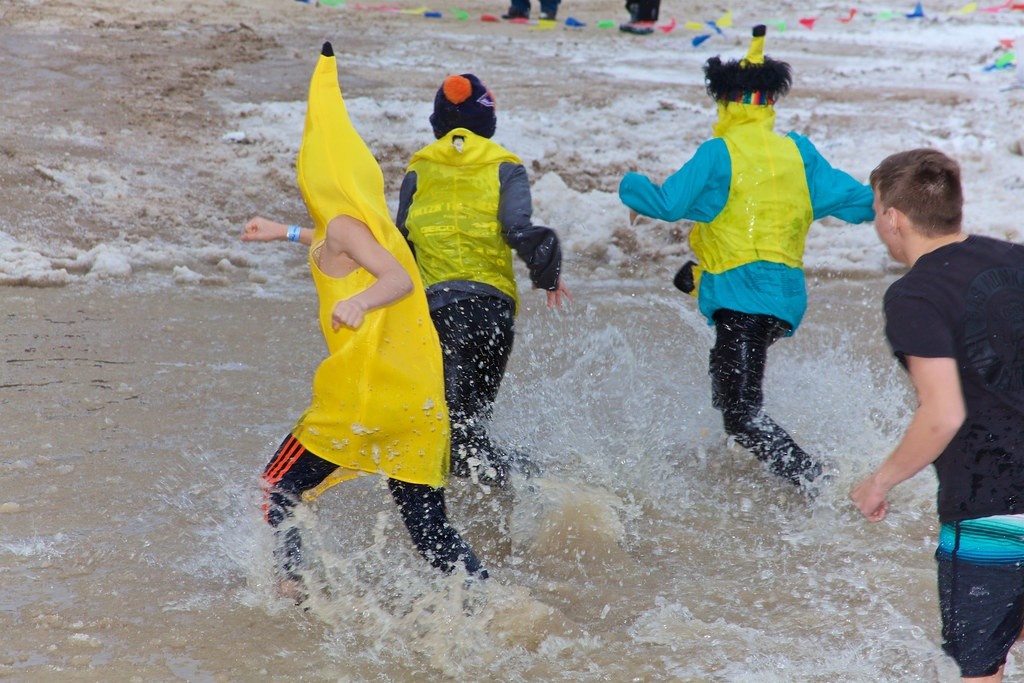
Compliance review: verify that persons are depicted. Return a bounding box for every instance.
[393,73,575,499]
[617,0,659,35]
[618,56,876,496]
[501,0,561,21]
[849,147,1024,682]
[239,38,500,621]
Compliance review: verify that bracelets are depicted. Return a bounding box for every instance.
[287,224,302,242]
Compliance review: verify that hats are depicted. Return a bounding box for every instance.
[428,73,496,138]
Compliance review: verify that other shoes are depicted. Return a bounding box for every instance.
[621,21,654,35]
[538,10,557,20]
[502,9,530,21]
